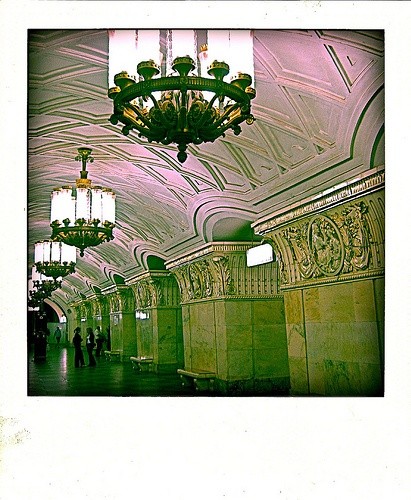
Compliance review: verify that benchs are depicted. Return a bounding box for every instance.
[105,351,120,364]
[178,369,215,393]
[130,357,153,372]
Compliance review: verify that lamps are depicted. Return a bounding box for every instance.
[50,147,117,257]
[28,227,77,312]
[107,30,256,164]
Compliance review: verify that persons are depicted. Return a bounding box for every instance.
[85,328,96,367]
[73,327,87,367]
[53,326,61,349]
[106,326,111,351]
[95,326,105,357]
